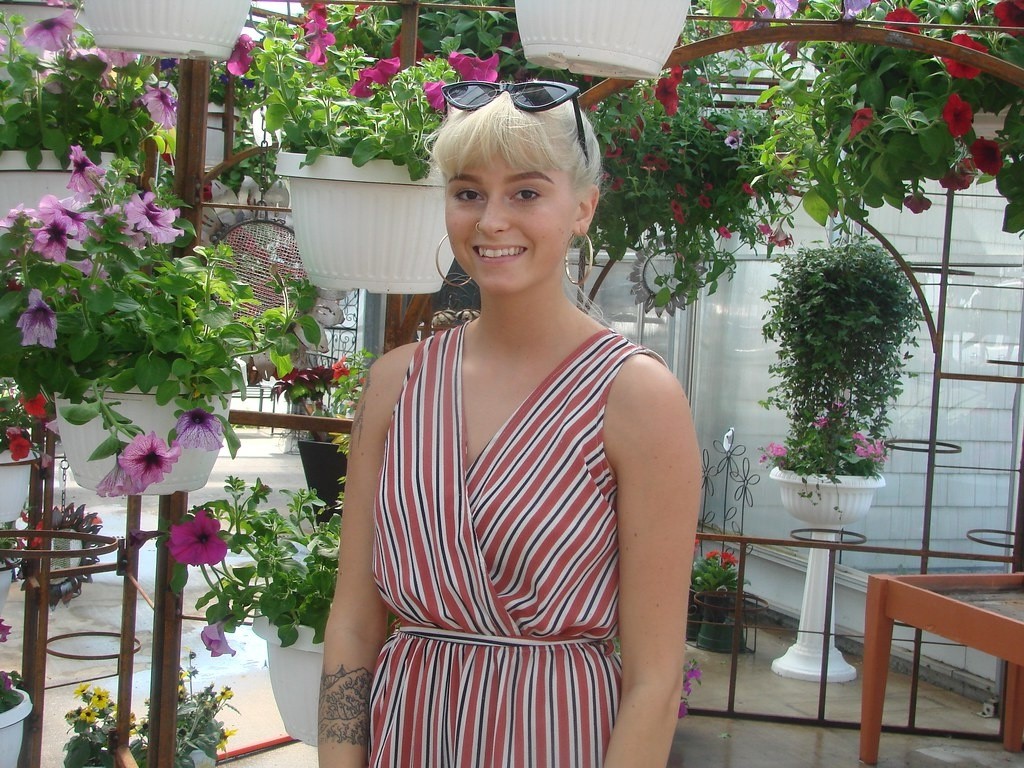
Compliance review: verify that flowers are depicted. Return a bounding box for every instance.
[269,347,386,442]
[128,473,348,658]
[0,501,53,583]
[0,376,57,482]
[136,646,243,760]
[225,0,502,183]
[0,619,25,714]
[0,0,180,174]
[757,399,899,524]
[704,0,1024,244]
[690,539,751,593]
[1,142,323,502]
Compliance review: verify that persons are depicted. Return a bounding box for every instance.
[315,80,704,768]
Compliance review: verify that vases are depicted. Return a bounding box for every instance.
[0,685,35,768]
[0,449,41,524]
[973,102,1013,142]
[694,591,748,654]
[274,153,458,295]
[175,745,219,768]
[686,587,704,643]
[296,439,349,527]
[251,608,328,748]
[0,149,129,265]
[0,558,20,614]
[49,356,250,497]
[767,465,889,685]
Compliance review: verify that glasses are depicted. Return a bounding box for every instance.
[440,81,590,168]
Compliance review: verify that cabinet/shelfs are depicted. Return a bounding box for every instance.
[858,570,1024,766]
